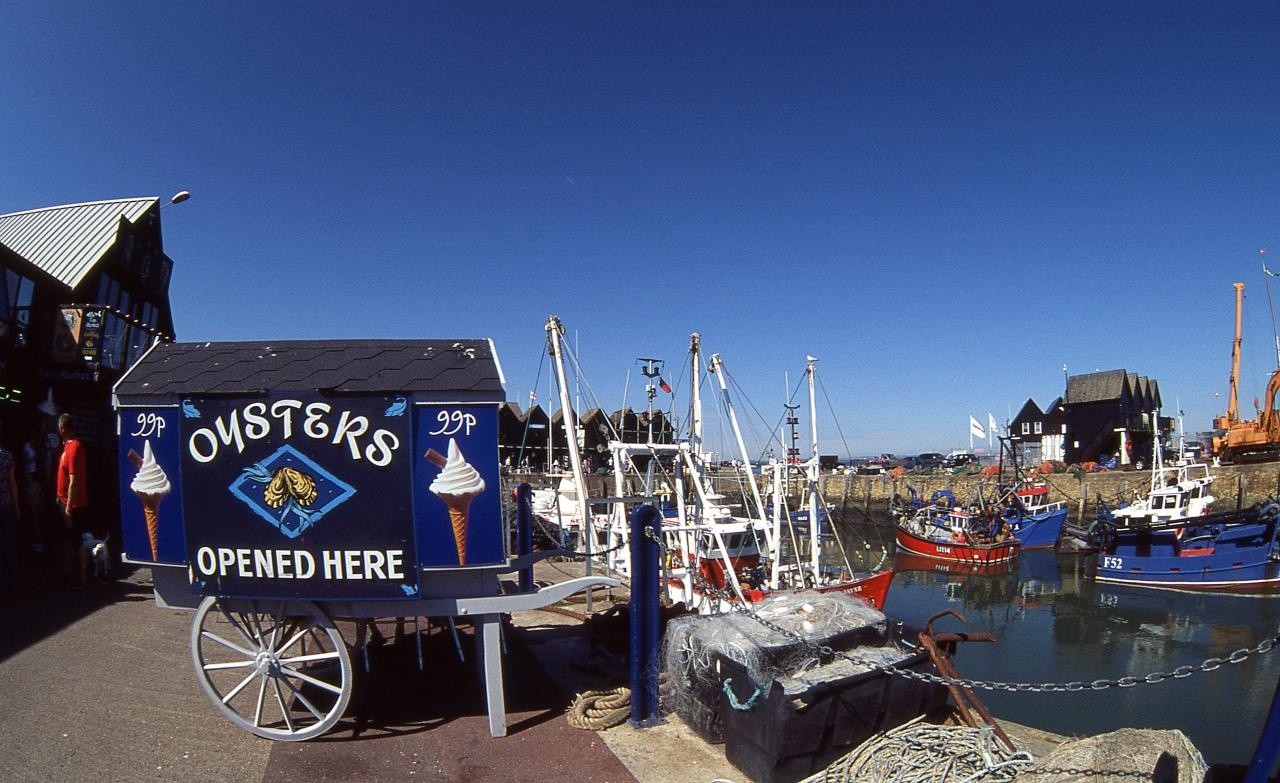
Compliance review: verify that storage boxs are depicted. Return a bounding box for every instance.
[717,623,953,783]
[672,591,890,743]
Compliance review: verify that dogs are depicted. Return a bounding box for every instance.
[81,533,113,586]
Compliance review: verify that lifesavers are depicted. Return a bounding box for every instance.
[1126,440,1132,450]
[1175,527,1184,540]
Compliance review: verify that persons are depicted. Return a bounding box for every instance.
[504,451,540,472]
[0,420,24,593]
[51,412,88,547]
[22,470,46,553]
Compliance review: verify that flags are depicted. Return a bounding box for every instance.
[658,377,672,393]
[971,417,985,438]
[990,414,998,432]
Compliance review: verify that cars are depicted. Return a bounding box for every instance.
[1129,443,1182,470]
[897,453,948,474]
[942,454,982,473]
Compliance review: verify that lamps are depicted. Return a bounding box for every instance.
[161,190,191,210]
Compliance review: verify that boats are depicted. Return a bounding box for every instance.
[996,487,1068,550]
[1090,507,1279,595]
[1110,409,1214,526]
[521,319,897,612]
[897,480,1019,562]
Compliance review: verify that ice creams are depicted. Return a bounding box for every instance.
[424,438,485,566]
[127,440,171,561]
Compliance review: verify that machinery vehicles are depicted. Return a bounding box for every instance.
[1216,281,1279,463]
